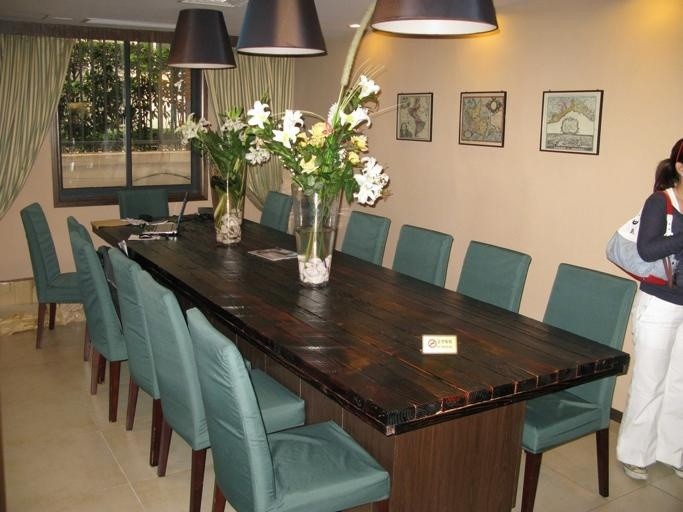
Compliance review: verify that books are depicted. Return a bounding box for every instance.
[246,245,299,262]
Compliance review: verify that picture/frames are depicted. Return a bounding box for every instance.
[457,90,506,148]
[538,88,603,155]
[395,92,434,143]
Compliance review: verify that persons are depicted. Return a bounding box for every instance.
[615,137,682,481]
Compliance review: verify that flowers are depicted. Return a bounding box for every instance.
[240,0,409,267]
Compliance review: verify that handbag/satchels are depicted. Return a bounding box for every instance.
[605,190,673,286]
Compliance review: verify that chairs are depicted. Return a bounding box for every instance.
[118,186,170,216]
[341,210,392,268]
[520,262,639,511]
[70,232,127,424]
[260,191,294,232]
[67,215,118,384]
[108,248,250,468]
[136,271,305,512]
[392,224,455,288]
[18,203,84,350]
[455,241,533,314]
[185,306,391,512]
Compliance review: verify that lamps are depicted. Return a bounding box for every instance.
[371,1,498,40]
[233,1,326,56]
[166,9,237,70]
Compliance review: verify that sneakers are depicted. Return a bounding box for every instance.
[623,463,648,480]
[672,466,683,478]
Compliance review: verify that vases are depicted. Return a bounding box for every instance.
[290,181,343,284]
[203,174,247,246]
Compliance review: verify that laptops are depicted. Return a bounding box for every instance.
[142,192,189,235]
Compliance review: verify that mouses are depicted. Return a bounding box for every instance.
[139,234,152,238]
